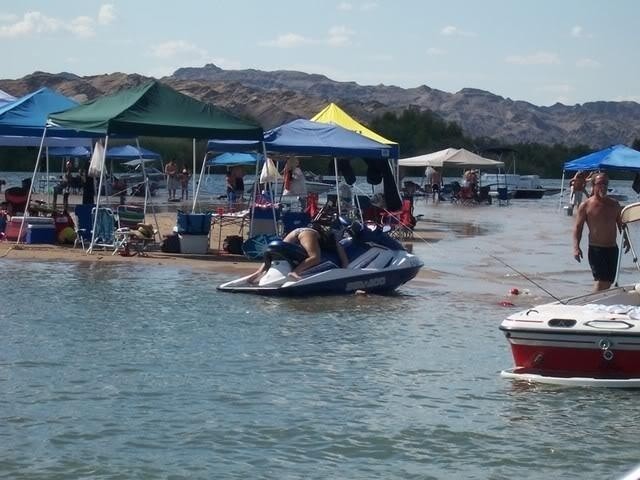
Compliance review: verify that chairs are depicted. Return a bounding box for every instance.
[73,204,152,258]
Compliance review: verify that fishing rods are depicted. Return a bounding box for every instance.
[466,242,567,304]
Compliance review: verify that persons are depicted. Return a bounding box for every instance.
[242,222,360,282]
[461,169,478,200]
[235,163,248,203]
[571,173,631,295]
[177,167,192,199]
[584,170,601,196]
[568,171,588,208]
[164,156,177,199]
[429,166,443,203]
[226,166,238,209]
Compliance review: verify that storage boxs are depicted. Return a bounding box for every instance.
[26,224,56,243]
[176,209,212,254]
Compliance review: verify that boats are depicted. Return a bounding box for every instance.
[215,212,425,297]
[243,164,336,197]
[496,201,640,389]
[118,157,193,191]
[473,144,567,198]
